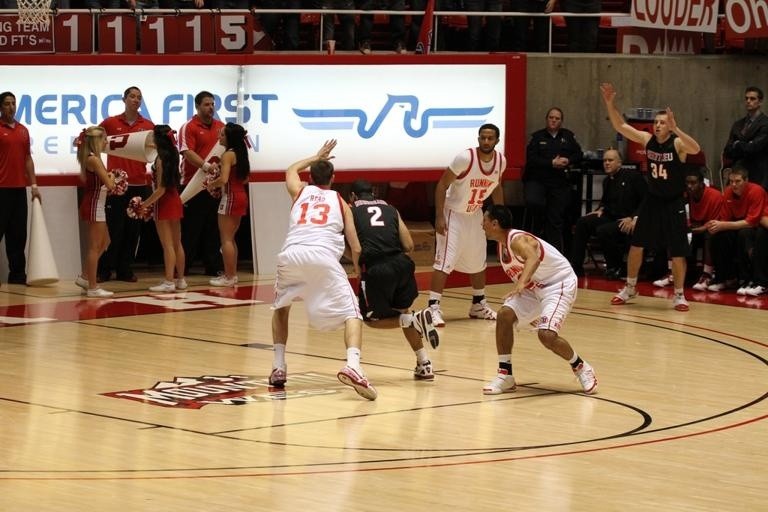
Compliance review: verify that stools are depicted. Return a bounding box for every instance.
[582,236,608,272]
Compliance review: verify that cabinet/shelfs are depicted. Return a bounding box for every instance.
[577,158,641,217]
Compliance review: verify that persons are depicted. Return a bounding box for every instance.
[343,179,441,380]
[127,0,609,57]
[722,86,768,184]
[477,203,599,398]
[520,108,585,275]
[2,91,42,286]
[427,122,504,327]
[654,167,768,295]
[599,82,701,313]
[592,149,646,281]
[73,85,253,298]
[268,137,380,400]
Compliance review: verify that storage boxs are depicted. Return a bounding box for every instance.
[397,217,437,268]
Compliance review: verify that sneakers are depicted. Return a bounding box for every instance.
[7,262,239,298]
[337,364,377,400]
[469,298,497,321]
[573,359,596,392]
[598,265,768,311]
[426,302,445,328]
[269,369,286,388]
[481,368,516,395]
[414,362,433,376]
[414,308,440,349]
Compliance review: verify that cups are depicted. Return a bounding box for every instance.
[597,149,604,158]
[326,40,336,54]
[637,108,652,118]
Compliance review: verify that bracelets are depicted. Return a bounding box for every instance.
[31,181,40,191]
